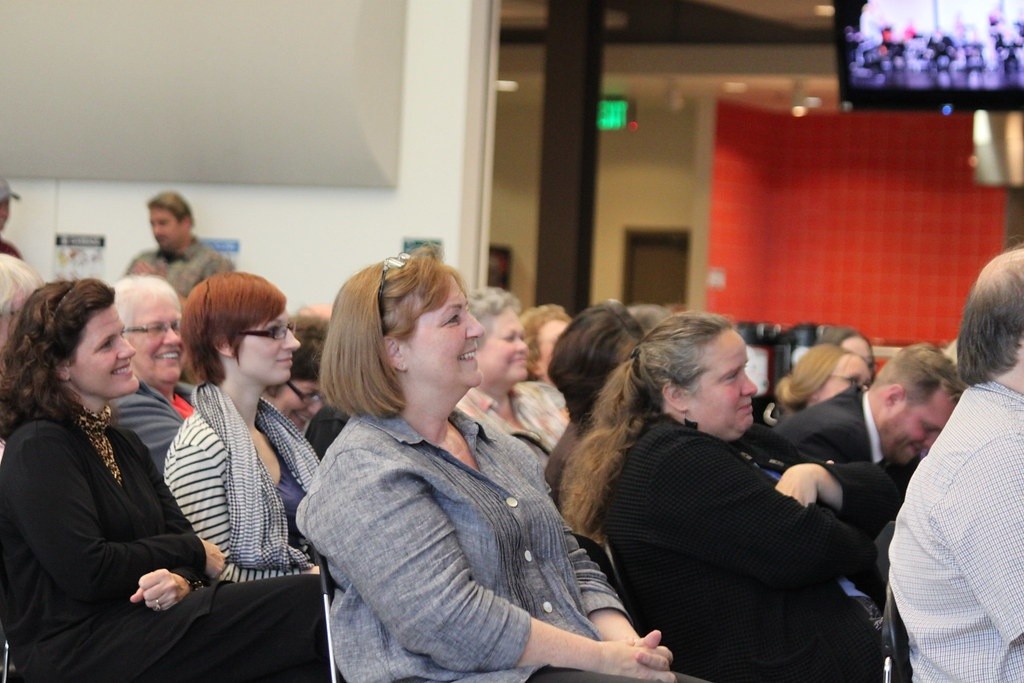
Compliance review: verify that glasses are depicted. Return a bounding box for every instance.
[238,322,298,339]
[829,373,858,386]
[378,252,412,306]
[124,320,183,338]
[287,380,326,405]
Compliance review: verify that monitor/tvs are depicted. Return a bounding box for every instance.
[831,0,1024,112]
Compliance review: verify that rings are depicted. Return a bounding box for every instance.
[153,599,162,612]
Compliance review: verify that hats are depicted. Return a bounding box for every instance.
[0,177,20,201]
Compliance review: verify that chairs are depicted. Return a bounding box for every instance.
[322,534,633,683]
[879,583,912,683]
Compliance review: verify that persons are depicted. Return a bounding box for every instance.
[856,7,1024,88]
[0,173,965,683]
[890,241,1024,683]
